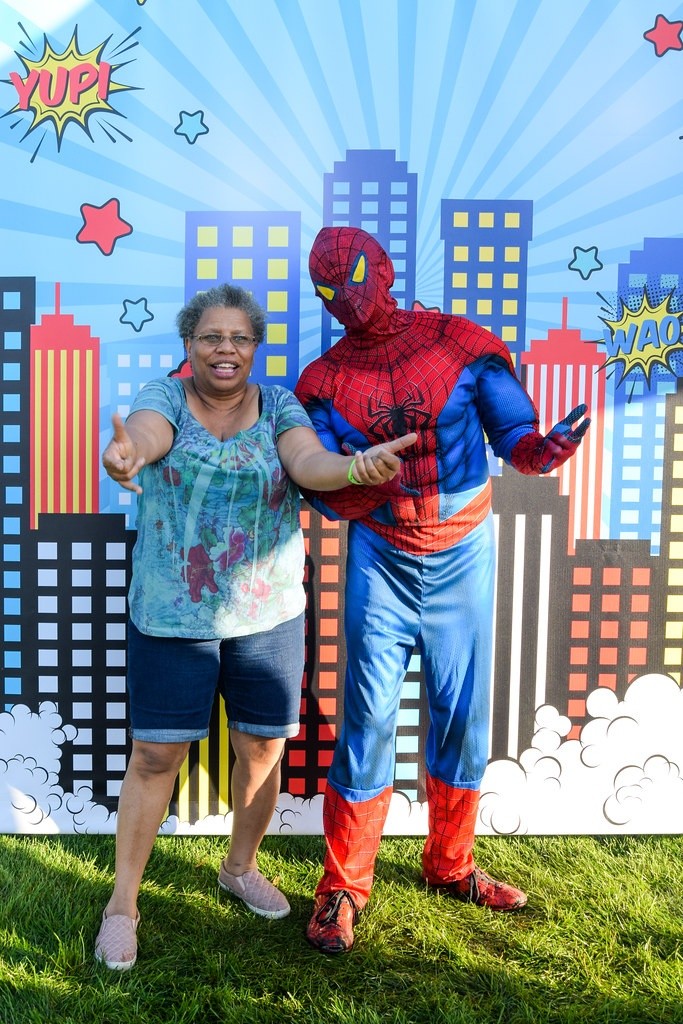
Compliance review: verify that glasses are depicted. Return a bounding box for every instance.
[189,333,256,349]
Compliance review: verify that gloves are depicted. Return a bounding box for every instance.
[535,404,591,473]
[342,442,421,502]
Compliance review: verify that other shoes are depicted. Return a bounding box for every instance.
[94,905,141,970]
[217,858,290,919]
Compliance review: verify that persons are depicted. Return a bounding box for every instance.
[92,286,418,972]
[291,226,591,955]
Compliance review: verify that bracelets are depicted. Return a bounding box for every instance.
[348,459,364,485]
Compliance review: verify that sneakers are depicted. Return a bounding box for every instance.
[430,862,528,912]
[308,888,360,952]
